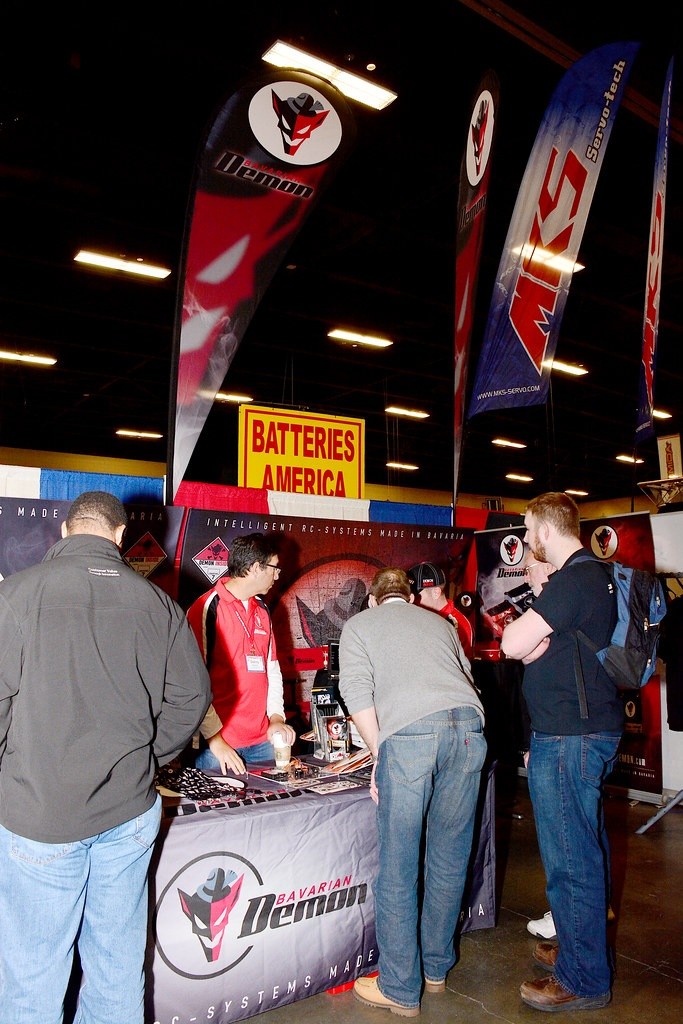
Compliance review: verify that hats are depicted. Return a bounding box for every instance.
[323,578,367,631]
[407,561,446,596]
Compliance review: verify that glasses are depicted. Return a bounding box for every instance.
[524,562,542,575]
[252,559,282,575]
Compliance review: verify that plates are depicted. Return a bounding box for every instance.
[210,777,244,789]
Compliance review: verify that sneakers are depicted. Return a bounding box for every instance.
[608,907,615,920]
[527,910,558,940]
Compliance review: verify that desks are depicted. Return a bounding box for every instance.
[146,753,498,1024]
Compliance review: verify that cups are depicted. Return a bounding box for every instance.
[272,734,292,767]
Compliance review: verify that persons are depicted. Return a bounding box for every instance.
[500,491,625,1010]
[187,532,296,776]
[338,561,487,1016]
[0,493,211,1024]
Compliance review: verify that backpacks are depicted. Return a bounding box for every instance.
[562,555,668,720]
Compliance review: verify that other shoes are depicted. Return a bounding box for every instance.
[520,974,611,1011]
[533,941,560,967]
[352,975,420,1018]
[421,974,446,993]
